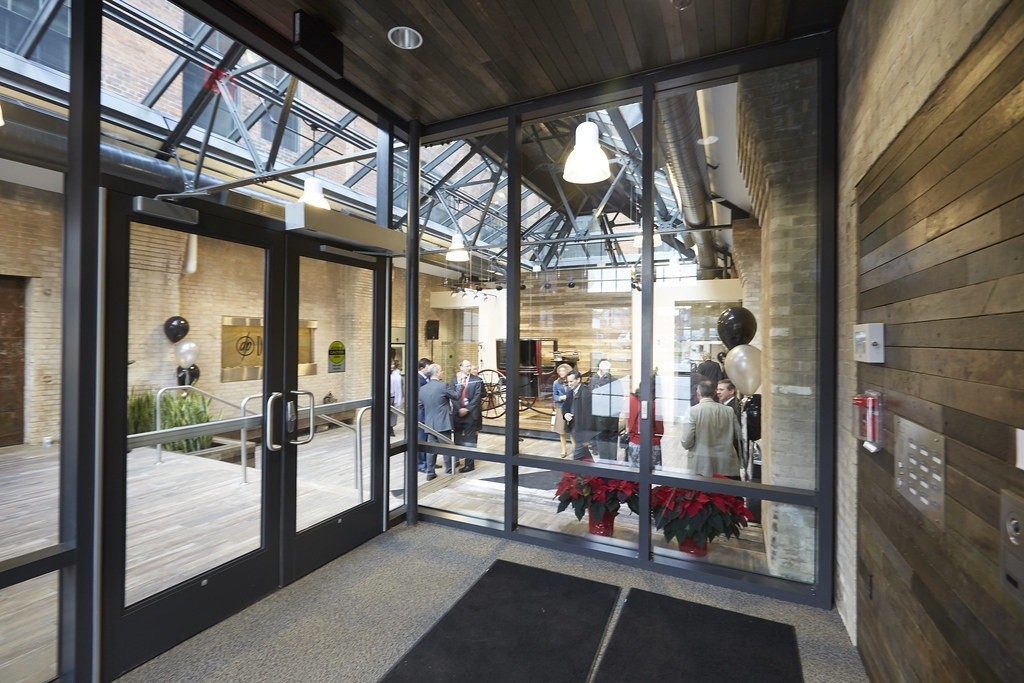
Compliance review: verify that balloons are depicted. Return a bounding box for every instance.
[164,316,189,343]
[175,340,199,368]
[177,364,200,391]
[717,307,757,350]
[725,344,761,395]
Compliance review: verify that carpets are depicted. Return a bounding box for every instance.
[590,588,803,683]
[374,560,622,683]
[392,470,562,497]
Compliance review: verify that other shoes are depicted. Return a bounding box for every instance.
[561,453,567,458]
[421,468,427,473]
[427,474,437,481]
[435,464,442,468]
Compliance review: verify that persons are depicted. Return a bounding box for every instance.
[628,382,664,471]
[562,370,596,461]
[419,364,464,481]
[553,364,576,457]
[698,352,724,381]
[418,358,442,474]
[450,360,487,473]
[390,359,403,437]
[681,380,743,481]
[590,361,619,461]
[717,379,741,425]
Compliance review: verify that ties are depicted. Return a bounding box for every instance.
[461,376,467,408]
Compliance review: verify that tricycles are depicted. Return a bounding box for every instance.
[476,337,592,419]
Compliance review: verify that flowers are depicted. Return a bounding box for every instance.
[651,472,753,548]
[552,458,640,524]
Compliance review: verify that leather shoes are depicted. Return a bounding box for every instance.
[458,466,474,473]
[455,462,460,468]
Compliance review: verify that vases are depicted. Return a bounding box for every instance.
[678,537,709,556]
[588,505,614,537]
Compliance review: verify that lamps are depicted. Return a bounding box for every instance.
[451,286,499,303]
[568,282,574,287]
[563,112,612,184]
[520,285,526,290]
[298,125,332,210]
[446,197,470,262]
[710,192,726,203]
[496,286,502,290]
[544,283,551,288]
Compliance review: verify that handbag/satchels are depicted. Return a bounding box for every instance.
[620,433,631,448]
